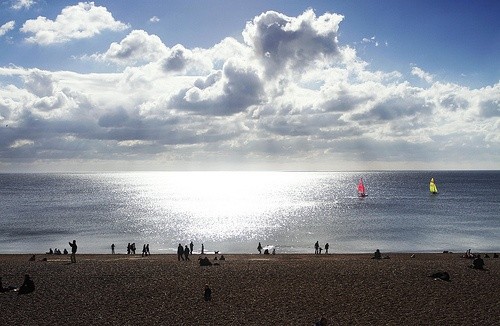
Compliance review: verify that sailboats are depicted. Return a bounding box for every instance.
[357,177,369,197]
[430,178,439,194]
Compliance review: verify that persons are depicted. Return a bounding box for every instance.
[204,284,212,301]
[190,242,193,254]
[46,248,68,255]
[111,244,115,254]
[204,257,209,262]
[177,244,190,261]
[431,272,450,281]
[127,243,136,254]
[374,249,381,259]
[29,255,35,261]
[315,241,329,254]
[20,274,35,293]
[142,244,151,257]
[202,244,204,254]
[69,240,77,263]
[257,242,276,255]
[473,255,484,269]
[462,249,499,258]
